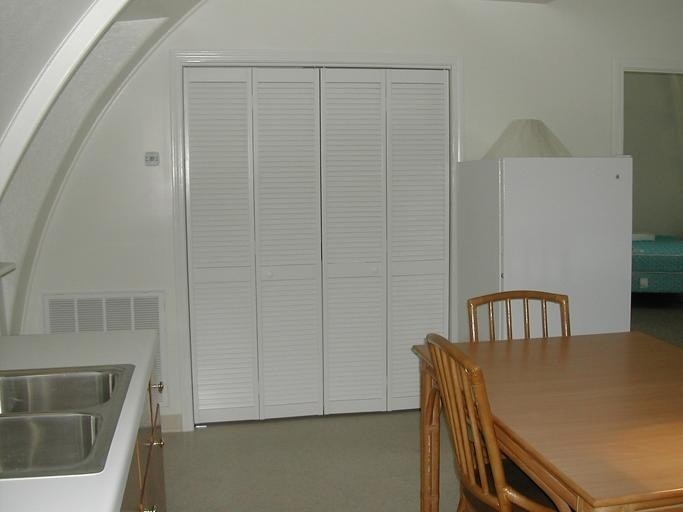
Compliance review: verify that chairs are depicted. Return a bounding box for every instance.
[424,291,571,512]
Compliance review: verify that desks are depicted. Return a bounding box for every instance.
[411,330,681,512]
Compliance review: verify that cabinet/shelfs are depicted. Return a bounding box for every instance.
[114,365,167,510]
[181,66,451,424]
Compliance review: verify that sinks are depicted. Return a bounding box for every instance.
[1,413,110,477]
[1,364,136,412]
